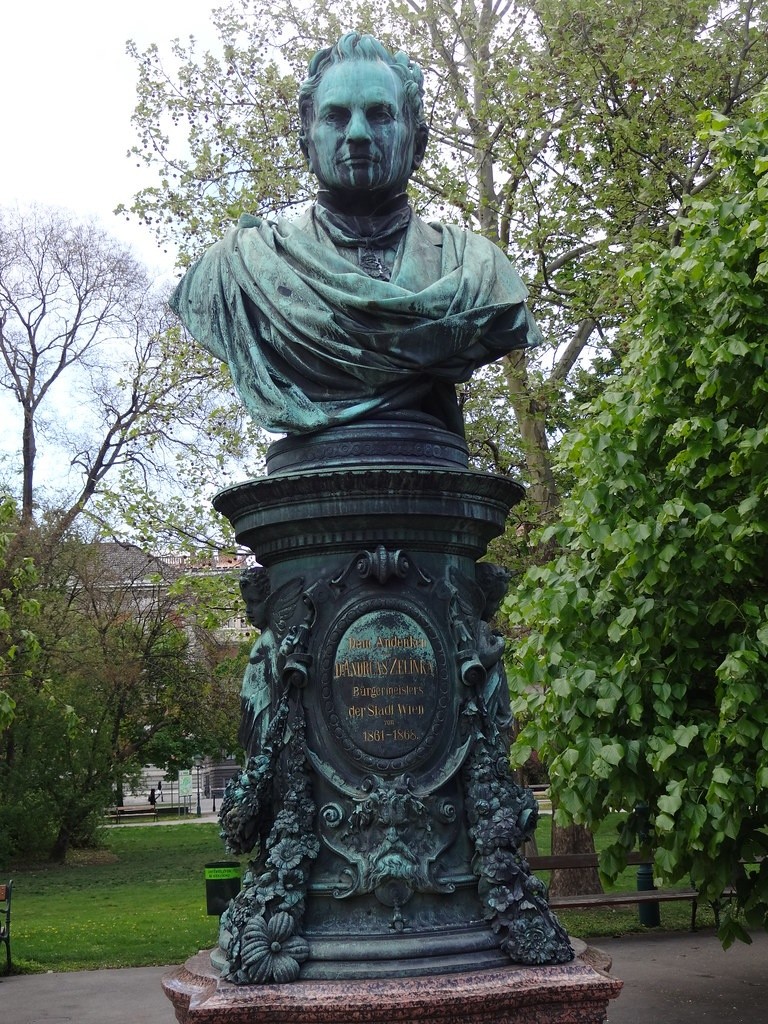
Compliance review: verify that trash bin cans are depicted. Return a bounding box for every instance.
[205,862,242,914]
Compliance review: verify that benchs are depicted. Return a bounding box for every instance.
[102,805,159,824]
[0,878,13,980]
[526,851,736,932]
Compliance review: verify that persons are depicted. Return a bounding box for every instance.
[150,789,156,805]
[158,782,161,790]
[168,33,544,435]
